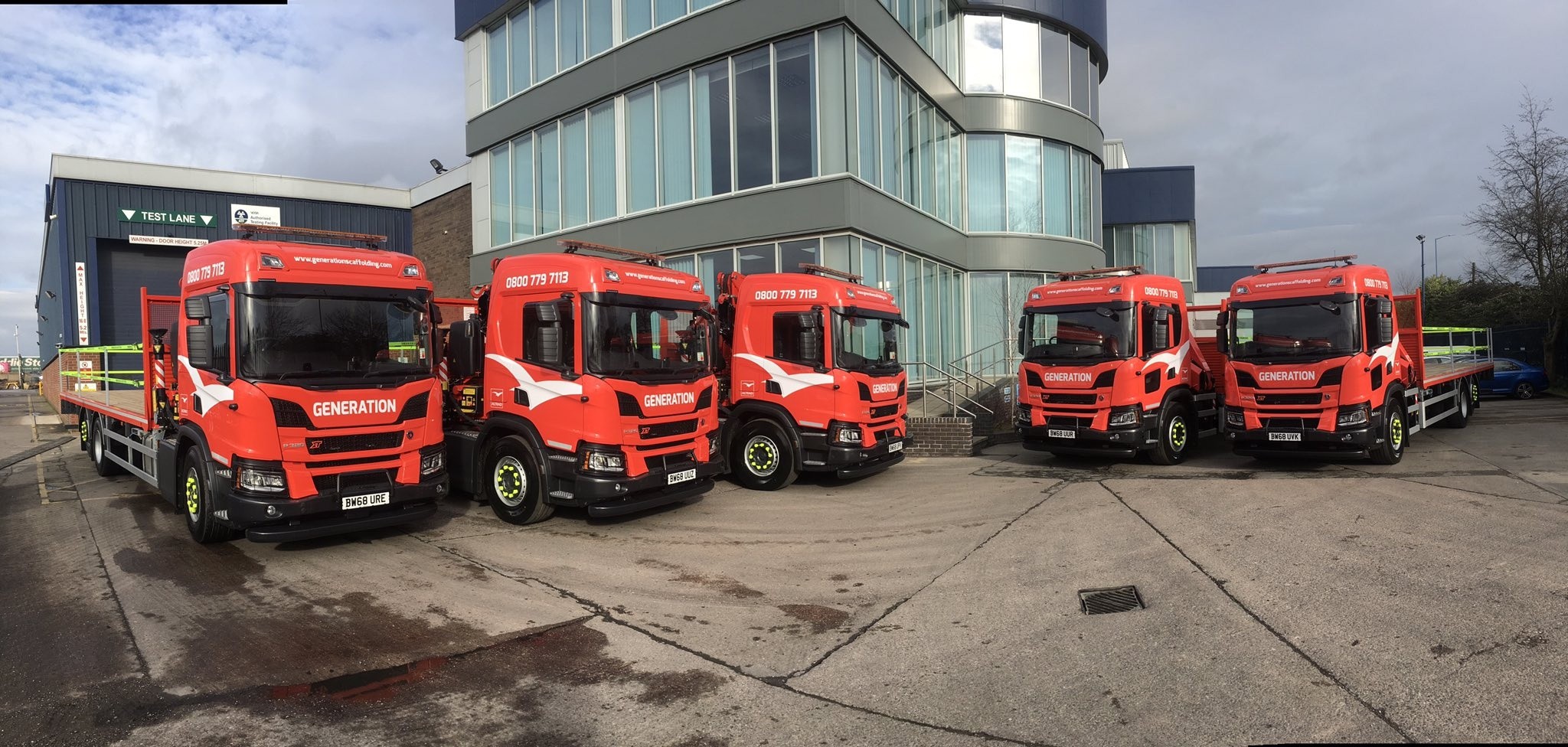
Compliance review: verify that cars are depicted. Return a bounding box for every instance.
[1448,358,1550,399]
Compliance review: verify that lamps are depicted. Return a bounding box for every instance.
[36,332,43,335]
[37,341,40,345]
[430,158,444,173]
[39,315,47,321]
[43,290,56,299]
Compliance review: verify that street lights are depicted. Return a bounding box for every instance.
[1416,234,1426,326]
[1435,234,1455,277]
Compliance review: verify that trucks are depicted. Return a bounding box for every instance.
[1016,266,1223,463]
[435,240,723,526]
[58,225,447,543]
[710,265,911,492]
[1217,256,1495,466]
